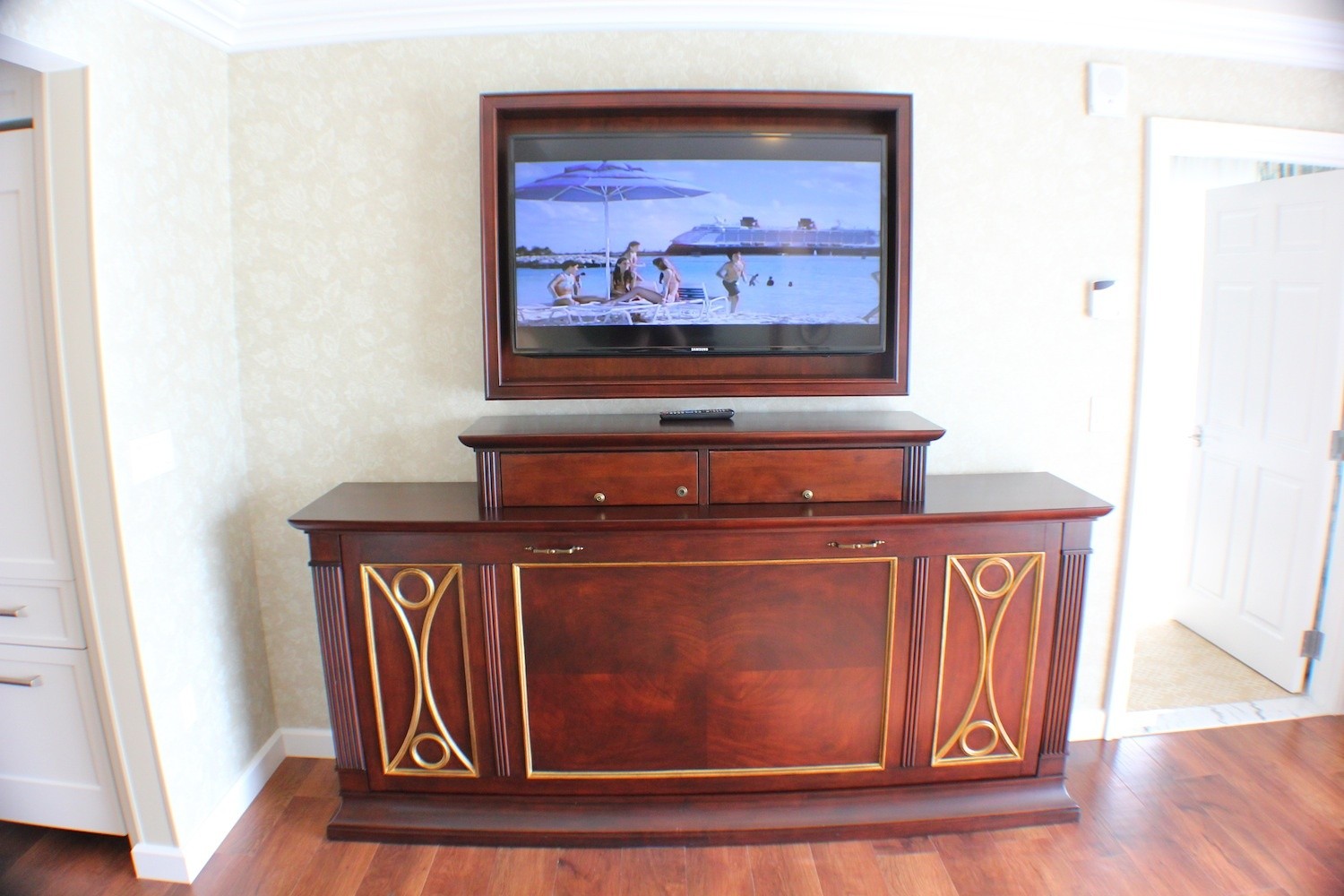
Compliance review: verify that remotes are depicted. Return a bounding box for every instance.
[659,409,735,421]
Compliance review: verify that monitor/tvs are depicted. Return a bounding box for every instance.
[508,132,885,357]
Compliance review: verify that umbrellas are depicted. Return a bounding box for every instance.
[514,160,712,301]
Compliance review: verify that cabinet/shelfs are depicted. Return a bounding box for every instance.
[457,411,950,505]
[286,470,1117,852]
[0,118,128,839]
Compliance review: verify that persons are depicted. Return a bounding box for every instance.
[611,256,681,307]
[766,276,774,286]
[548,260,607,307]
[611,258,640,301]
[788,281,793,287]
[621,241,644,282]
[749,273,759,286]
[575,272,586,296]
[715,247,748,313]
